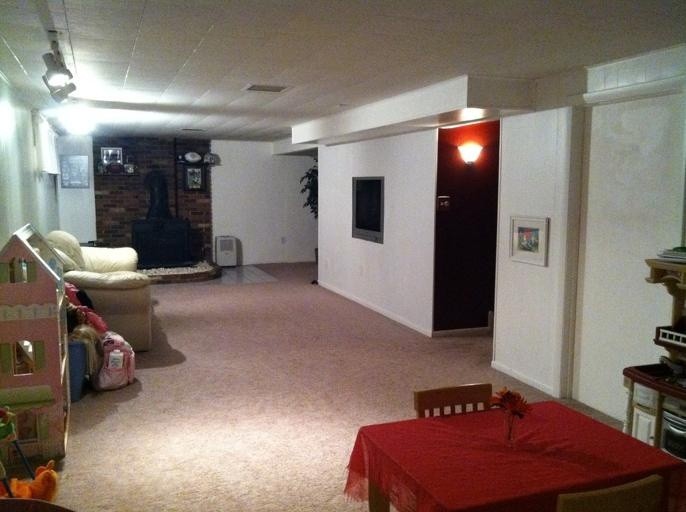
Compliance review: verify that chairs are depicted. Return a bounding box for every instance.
[413,383,493,418]
[555,473,664,512]
[45,229,152,352]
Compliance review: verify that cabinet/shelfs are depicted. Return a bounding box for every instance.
[621,259,685,463]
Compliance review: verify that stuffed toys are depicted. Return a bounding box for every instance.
[7,459,58,500]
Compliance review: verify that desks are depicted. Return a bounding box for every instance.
[359,399,686,511]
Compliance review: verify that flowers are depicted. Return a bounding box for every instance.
[490,385,534,440]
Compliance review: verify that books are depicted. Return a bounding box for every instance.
[656,246,686,263]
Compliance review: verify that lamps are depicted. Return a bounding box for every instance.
[42,53,76,104]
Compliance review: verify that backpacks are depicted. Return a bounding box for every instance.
[92,329,136,393]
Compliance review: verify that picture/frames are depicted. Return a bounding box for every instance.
[509,215,550,268]
[100,147,123,165]
[183,164,206,192]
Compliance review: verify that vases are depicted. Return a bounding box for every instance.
[503,414,519,448]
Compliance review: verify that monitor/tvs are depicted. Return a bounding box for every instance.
[351,176,384,245]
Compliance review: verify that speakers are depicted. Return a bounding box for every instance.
[215,236,238,267]
